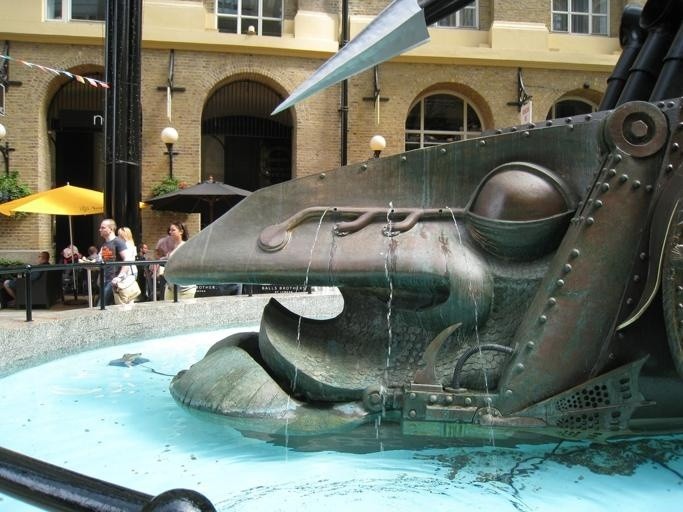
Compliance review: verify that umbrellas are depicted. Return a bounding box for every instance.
[0,181,152,305]
[144,176,253,225]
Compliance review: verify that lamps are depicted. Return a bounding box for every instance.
[161,127,180,181]
[0,124,15,176]
[369,135,386,159]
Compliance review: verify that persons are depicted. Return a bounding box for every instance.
[58,219,197,306]
[3,251,50,308]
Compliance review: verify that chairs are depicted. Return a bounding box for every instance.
[17,268,102,308]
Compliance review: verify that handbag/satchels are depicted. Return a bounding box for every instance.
[111,273,141,305]
